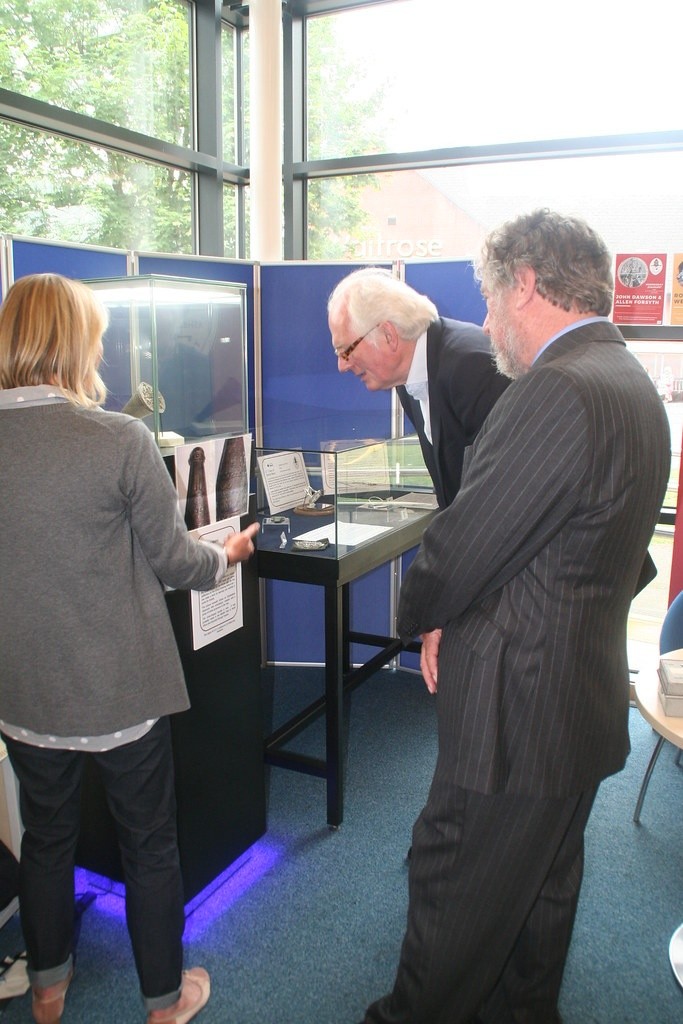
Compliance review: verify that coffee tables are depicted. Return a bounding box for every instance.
[633,646,683,822]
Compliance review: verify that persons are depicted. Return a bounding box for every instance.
[327,267,658,601]
[364,205,671,1023]
[1,273,259,1024]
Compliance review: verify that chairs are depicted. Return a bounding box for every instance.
[659,589,683,656]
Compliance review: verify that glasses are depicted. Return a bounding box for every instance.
[336,323,380,361]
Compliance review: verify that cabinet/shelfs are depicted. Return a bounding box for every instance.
[69,275,268,920]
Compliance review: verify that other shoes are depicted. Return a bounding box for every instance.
[32,965,73,1024]
[146,967,211,1024]
